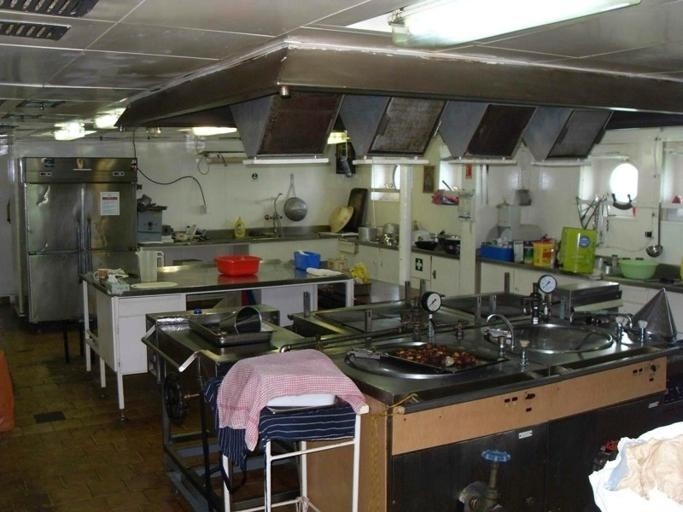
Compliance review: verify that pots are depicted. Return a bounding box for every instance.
[284,185,308,222]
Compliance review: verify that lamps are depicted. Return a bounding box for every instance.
[387,0,639,48]
[53,106,348,146]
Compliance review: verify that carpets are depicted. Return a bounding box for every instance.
[0,349,16,434]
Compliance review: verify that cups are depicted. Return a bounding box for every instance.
[93,268,111,285]
[135,250,164,282]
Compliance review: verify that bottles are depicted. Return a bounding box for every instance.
[523,239,534,265]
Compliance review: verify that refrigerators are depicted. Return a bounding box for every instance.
[16,156,145,323]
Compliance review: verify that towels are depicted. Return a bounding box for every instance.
[217,348,370,452]
[588,421,683,512]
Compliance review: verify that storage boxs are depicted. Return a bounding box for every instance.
[294,250,320,271]
[136,208,163,242]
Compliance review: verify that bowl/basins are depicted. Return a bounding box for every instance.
[213,254,263,276]
[329,205,354,233]
[617,259,660,280]
[415,239,438,250]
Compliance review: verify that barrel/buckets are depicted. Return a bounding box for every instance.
[560,226,597,274]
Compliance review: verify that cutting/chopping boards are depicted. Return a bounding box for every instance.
[345,188,367,233]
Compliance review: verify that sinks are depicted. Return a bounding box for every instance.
[251,235,332,243]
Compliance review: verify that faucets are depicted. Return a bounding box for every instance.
[485,314,515,347]
[274,192,282,207]
